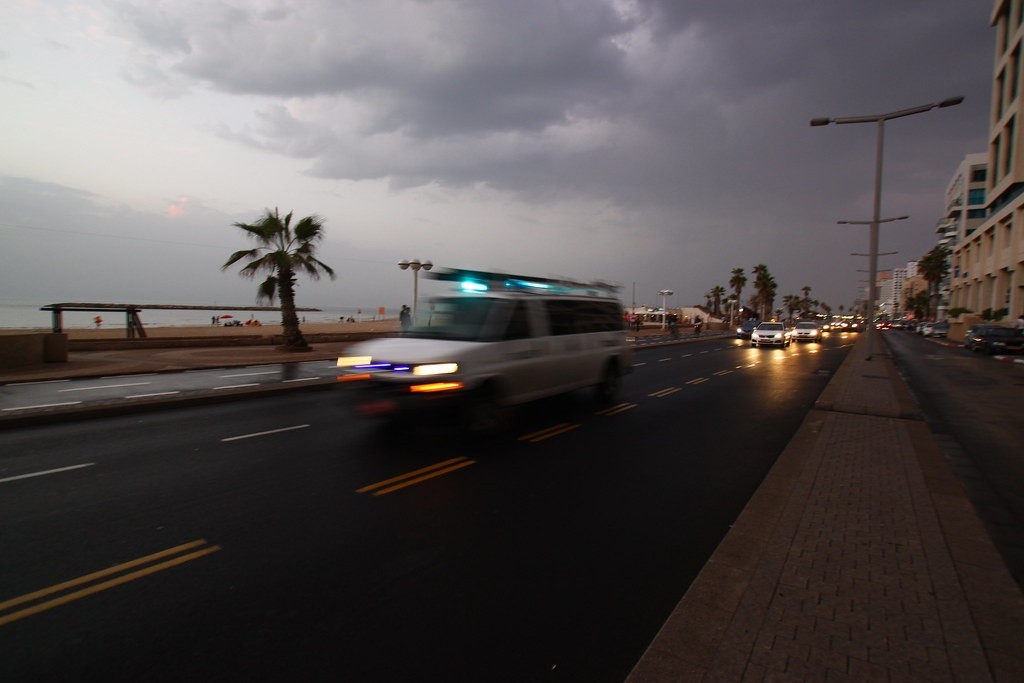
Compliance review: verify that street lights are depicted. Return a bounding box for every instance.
[808,94,967,361]
[658,288,673,330]
[728,300,738,326]
[398,259,433,318]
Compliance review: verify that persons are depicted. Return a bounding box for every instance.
[627,311,702,336]
[338,314,345,325]
[211,314,241,326]
[399,303,414,337]
[1016,314,1024,332]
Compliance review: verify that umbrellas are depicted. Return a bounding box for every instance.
[219,315,233,319]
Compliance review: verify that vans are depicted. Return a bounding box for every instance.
[336,269,626,430]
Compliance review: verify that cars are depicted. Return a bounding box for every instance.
[916,322,949,338]
[792,322,822,343]
[820,317,914,332]
[737,321,762,339]
[964,324,1024,355]
[751,322,791,348]
